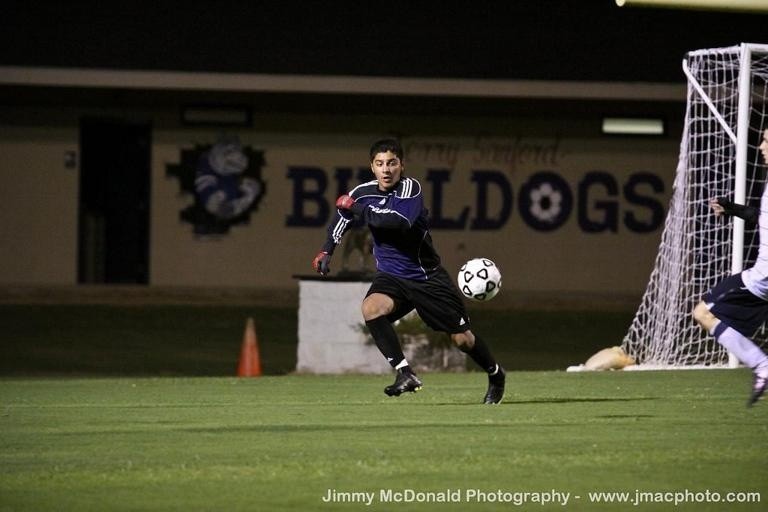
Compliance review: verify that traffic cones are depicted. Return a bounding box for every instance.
[239,318,262,377]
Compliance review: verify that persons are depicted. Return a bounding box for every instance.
[313,138,505,405]
[692,128,768,408]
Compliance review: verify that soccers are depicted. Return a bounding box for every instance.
[457,257,502,303]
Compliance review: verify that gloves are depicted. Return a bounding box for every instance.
[335,194,354,210]
[312,251,331,276]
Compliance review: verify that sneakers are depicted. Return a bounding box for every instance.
[483,364,506,405]
[384,372,423,396]
[747,372,768,403]
